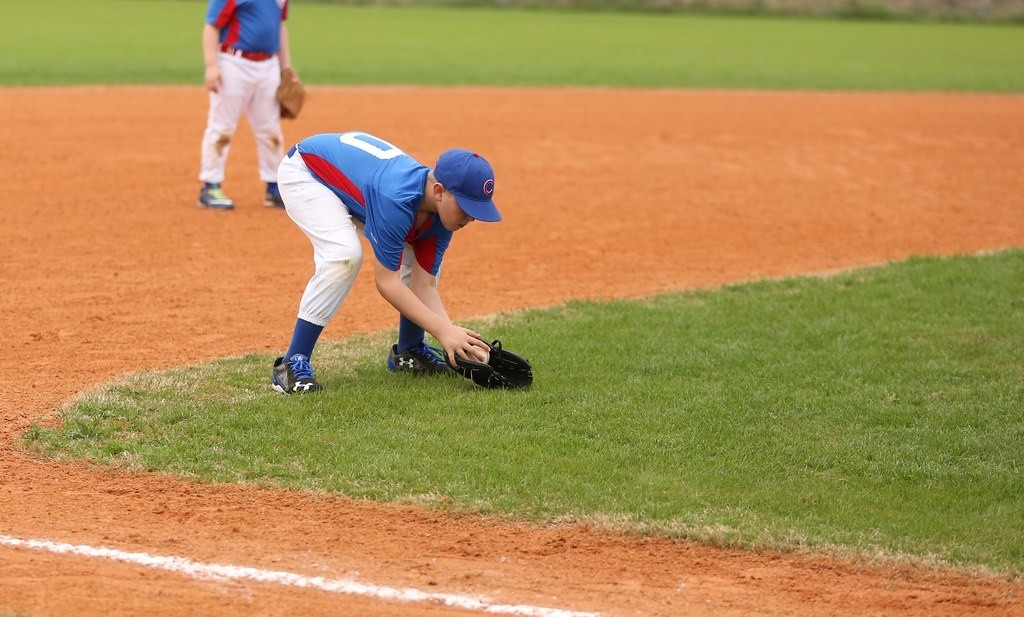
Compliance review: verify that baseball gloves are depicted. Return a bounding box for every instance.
[276,68,307,120]
[444,338,533,390]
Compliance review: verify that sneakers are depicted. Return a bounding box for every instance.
[271,353,323,396]
[262,182,285,209]
[385,337,457,377]
[196,182,234,209]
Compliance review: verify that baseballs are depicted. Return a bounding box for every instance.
[469,345,490,363]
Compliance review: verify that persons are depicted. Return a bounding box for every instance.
[196,0,306,210]
[272,131,532,396]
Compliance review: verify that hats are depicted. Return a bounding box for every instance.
[433,148,502,222]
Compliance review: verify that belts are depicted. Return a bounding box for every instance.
[286,143,297,158]
[221,44,272,61]
